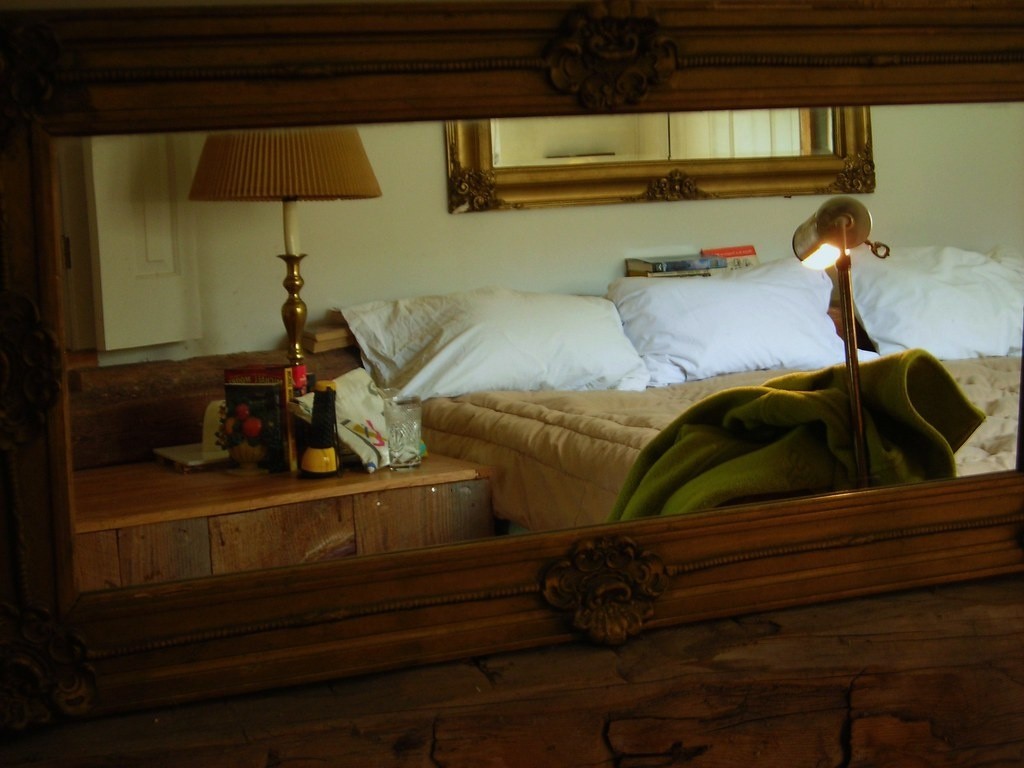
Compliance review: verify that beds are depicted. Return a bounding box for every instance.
[358,294,1022,532]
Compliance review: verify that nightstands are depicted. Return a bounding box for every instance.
[75,447,495,590]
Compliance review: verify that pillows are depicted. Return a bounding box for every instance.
[606,256,881,388]
[850,245,1024,361]
[331,287,652,401]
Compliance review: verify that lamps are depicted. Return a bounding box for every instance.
[188,124,383,366]
[796,193,890,493]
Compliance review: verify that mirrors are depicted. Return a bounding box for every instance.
[444,103,877,215]
[0,0,1024,733]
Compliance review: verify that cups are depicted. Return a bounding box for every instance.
[384,395,422,471]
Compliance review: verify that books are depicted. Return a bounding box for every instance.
[625,245,759,277]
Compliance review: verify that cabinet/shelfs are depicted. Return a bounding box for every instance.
[55,135,205,353]
